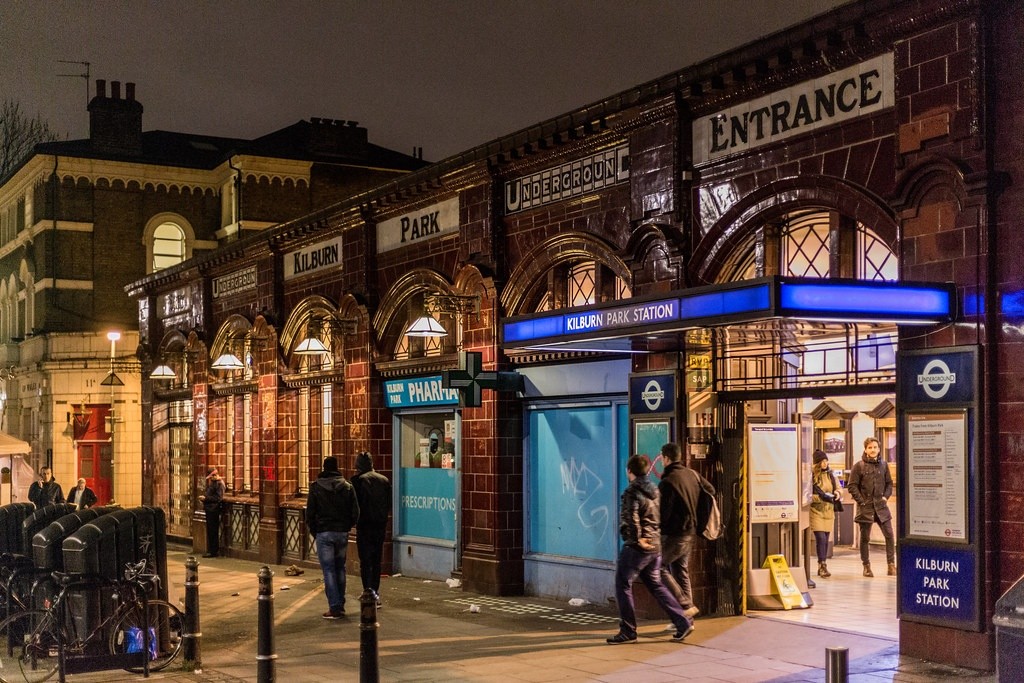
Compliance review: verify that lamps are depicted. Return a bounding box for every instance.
[145,346,200,379]
[100,359,150,386]
[215,330,270,370]
[72,393,93,430]
[292,308,358,354]
[403,283,481,336]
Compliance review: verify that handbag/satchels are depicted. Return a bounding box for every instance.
[689,469,726,540]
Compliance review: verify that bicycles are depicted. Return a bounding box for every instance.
[0,558,185,683]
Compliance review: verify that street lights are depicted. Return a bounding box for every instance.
[105,331,123,504]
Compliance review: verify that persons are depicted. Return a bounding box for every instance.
[28,467,65,509]
[67,477,97,510]
[352,451,390,607]
[305,456,360,619]
[809,448,843,578]
[198,468,226,558]
[607,456,695,645]
[657,443,715,628]
[848,437,896,577]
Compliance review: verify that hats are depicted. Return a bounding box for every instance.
[355,452,373,472]
[205,467,219,479]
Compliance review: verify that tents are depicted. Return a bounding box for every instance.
[0,431,32,503]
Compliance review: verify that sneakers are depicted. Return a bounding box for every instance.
[607,629,638,645]
[673,622,694,640]
[322,611,345,620]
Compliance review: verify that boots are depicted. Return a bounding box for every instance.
[818,562,831,578]
[863,563,874,577]
[888,565,896,576]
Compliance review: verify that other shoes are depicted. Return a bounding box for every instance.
[202,552,219,557]
